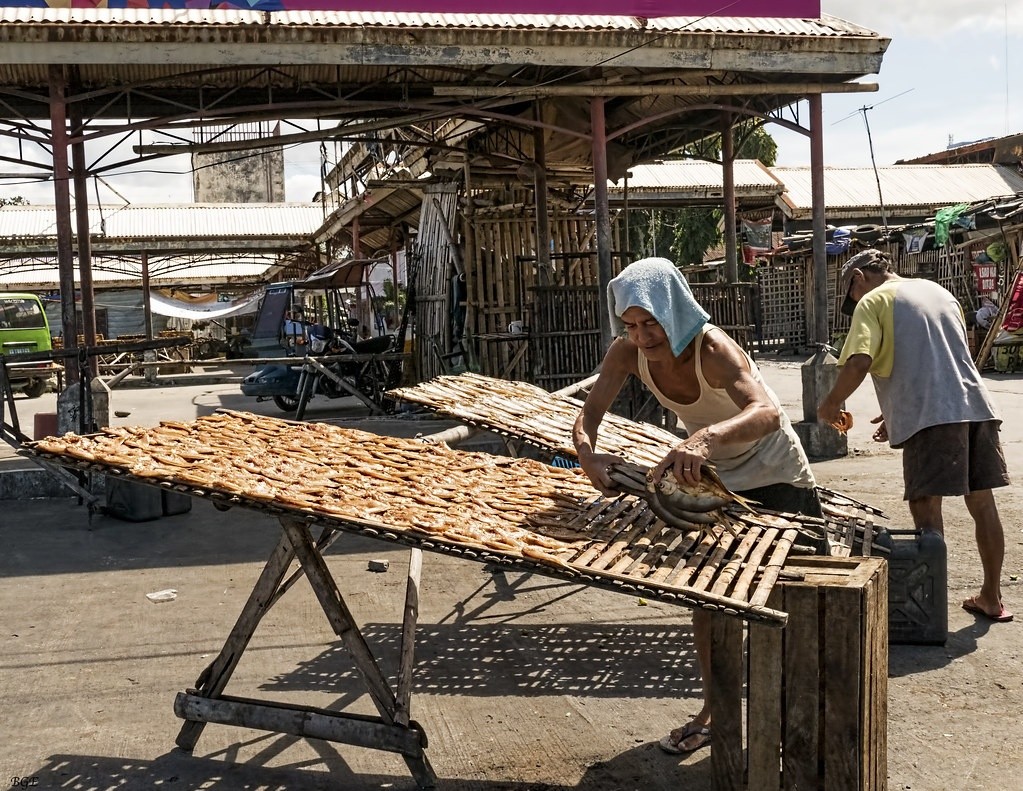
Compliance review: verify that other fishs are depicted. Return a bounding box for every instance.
[385,372,861,525]
[25,408,763,556]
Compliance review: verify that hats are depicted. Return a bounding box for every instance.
[840,249,881,315]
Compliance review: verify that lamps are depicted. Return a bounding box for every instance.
[517,126,543,187]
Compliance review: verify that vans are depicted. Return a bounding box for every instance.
[0,293,54,399]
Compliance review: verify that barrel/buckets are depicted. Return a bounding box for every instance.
[848,527,948,647]
[164,491,193,517]
[103,474,164,523]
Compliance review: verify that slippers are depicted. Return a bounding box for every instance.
[659,718,711,755]
[963,597,1013,621]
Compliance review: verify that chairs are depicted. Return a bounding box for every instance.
[433,340,471,376]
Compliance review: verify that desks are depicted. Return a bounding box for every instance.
[471,331,529,381]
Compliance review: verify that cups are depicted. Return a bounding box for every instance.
[508,320,523,335]
[231,326,236,334]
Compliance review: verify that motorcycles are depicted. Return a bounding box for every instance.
[284,316,399,421]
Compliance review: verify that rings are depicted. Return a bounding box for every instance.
[684,467,691,471]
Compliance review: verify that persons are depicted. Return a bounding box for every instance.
[571,256,833,755]
[818,249,1011,618]
